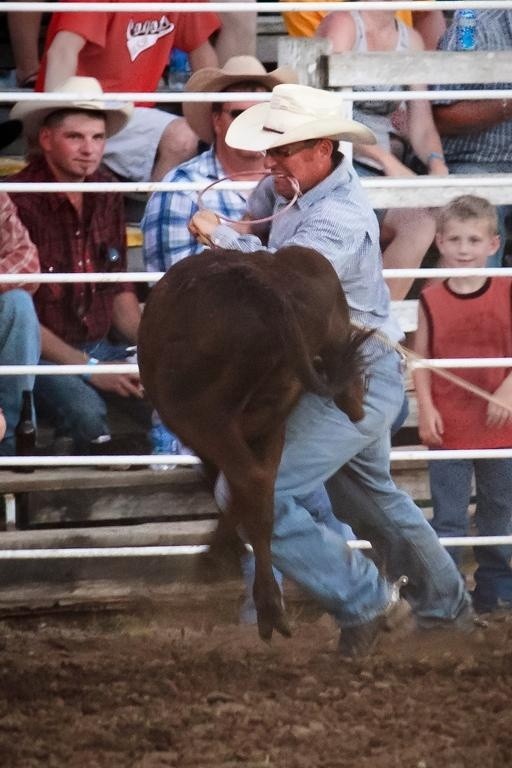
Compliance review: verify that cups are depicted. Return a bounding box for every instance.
[125,343,141,391]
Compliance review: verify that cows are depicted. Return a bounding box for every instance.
[132,246,380,647]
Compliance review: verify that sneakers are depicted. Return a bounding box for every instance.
[472,589,505,612]
[408,614,480,639]
[335,612,390,660]
[91,442,149,471]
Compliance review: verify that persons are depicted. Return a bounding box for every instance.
[2,1,511,466]
[410,190,510,621]
[186,83,477,676]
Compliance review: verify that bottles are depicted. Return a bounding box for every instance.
[150,407,177,469]
[454,10,477,52]
[168,48,192,90]
[13,389,37,475]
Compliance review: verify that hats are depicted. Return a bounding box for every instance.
[7,73,134,141]
[182,55,303,146]
[224,82,379,151]
